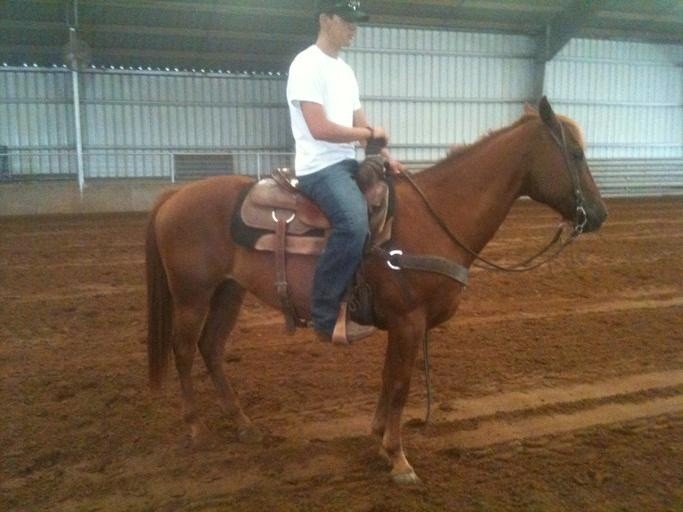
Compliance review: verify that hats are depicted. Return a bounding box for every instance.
[318,1,370,22]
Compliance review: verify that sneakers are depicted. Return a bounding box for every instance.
[314,318,375,344]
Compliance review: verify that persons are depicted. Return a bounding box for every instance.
[286,0,407,342]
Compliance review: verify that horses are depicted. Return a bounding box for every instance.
[143,94,609,489]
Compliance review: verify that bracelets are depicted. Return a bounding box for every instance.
[365,126,375,140]
